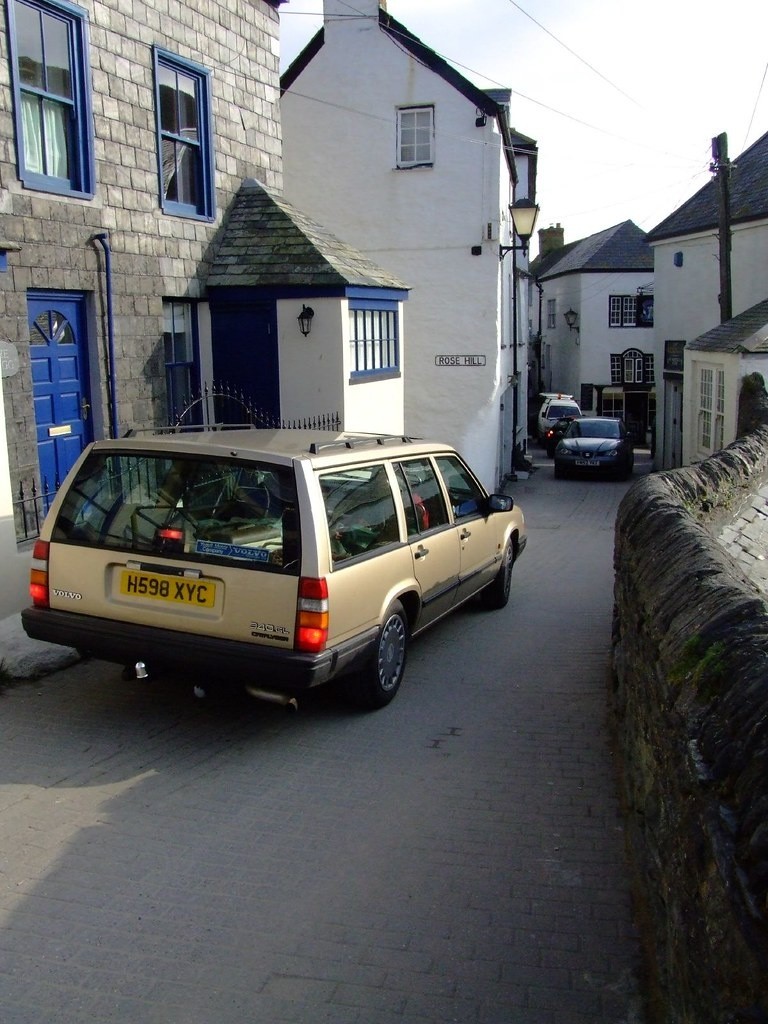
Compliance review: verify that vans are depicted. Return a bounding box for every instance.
[537,392,586,445]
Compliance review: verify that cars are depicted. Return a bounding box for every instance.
[19,426,530,710]
[545,418,576,457]
[554,416,636,480]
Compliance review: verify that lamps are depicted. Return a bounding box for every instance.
[507,375,519,388]
[499,196,541,262]
[563,307,580,333]
[297,304,315,338]
